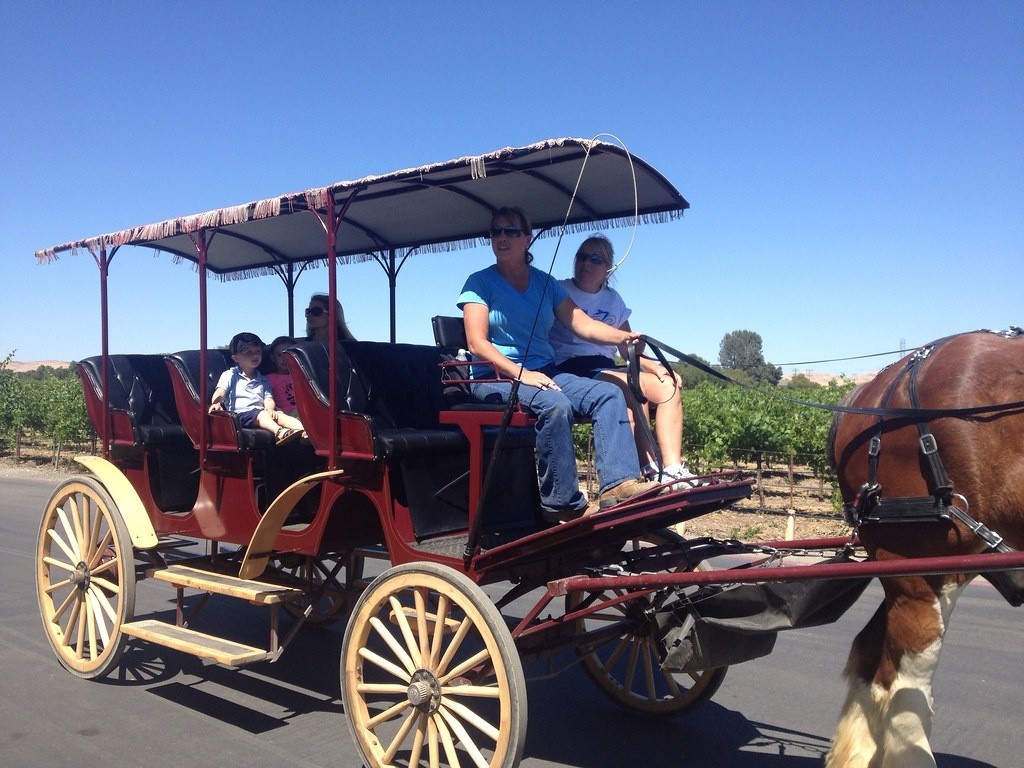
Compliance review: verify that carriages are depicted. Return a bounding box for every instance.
[33,133,1024,768]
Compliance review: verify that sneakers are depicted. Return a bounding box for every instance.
[648,471,693,495]
[665,462,709,487]
[561,502,600,523]
[600,479,663,508]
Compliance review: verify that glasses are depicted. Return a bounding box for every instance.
[489,226,524,238]
[575,252,609,266]
[305,307,328,317]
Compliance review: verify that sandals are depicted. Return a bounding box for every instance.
[275,427,304,445]
[301,431,310,444]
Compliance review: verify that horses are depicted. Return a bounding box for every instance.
[823,330,1024,768]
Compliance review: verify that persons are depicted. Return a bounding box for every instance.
[550,236,711,492]
[264,337,301,423]
[208,332,311,445]
[304,294,355,340]
[456,208,662,523]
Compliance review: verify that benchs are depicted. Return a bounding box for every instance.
[282,341,537,459]
[432,314,593,423]
[76,355,192,448]
[163,348,300,452]
